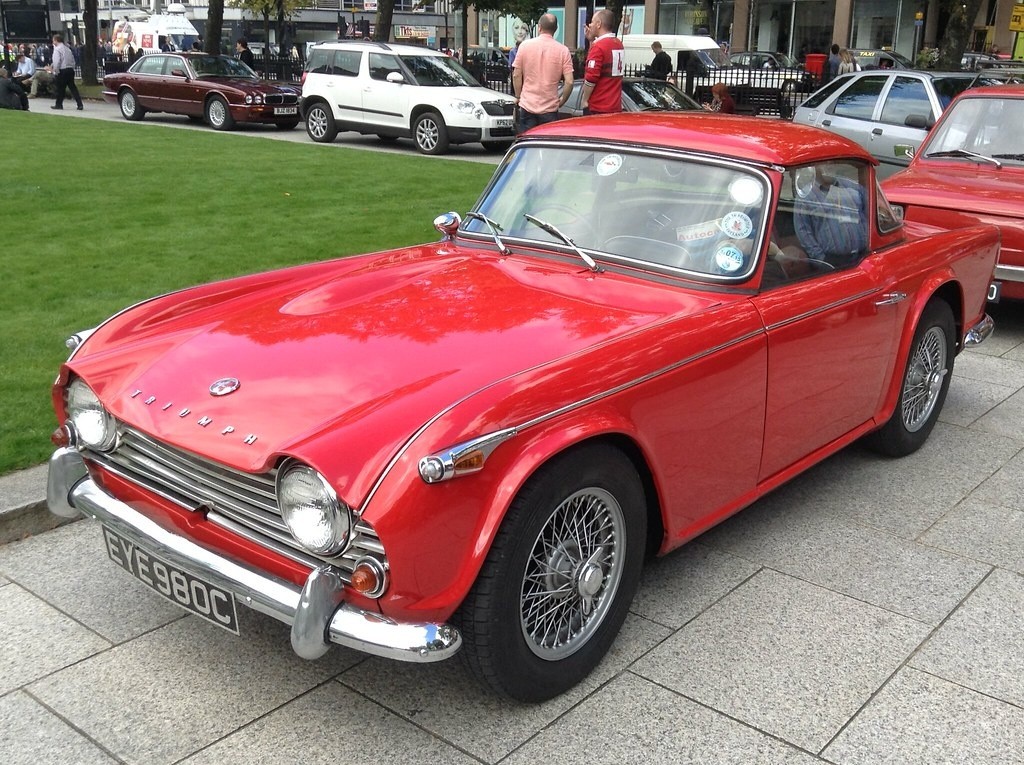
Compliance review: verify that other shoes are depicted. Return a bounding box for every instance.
[50,105,63,109]
[27,94,36,99]
[77,106,83,110]
[22,79,32,86]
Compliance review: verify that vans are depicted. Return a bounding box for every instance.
[616,34,728,94]
[111,15,203,62]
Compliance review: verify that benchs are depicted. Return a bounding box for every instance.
[620,200,863,273]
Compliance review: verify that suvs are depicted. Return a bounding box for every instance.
[302,40,518,155]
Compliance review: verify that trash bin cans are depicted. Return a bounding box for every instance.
[804,53,828,93]
[104,52,124,75]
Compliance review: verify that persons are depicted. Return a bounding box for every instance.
[761,57,775,68]
[880,60,894,69]
[701,81,735,114]
[162,35,204,52]
[512,18,529,42]
[581,9,625,116]
[643,41,683,110]
[237,38,255,71]
[508,13,574,134]
[823,43,861,85]
[794,159,870,273]
[0,33,110,110]
[989,44,1000,54]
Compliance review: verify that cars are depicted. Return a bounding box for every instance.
[46,110,1003,707]
[790,52,1024,184]
[882,85,1024,298]
[103,52,301,132]
[558,77,704,120]
[729,52,798,71]
